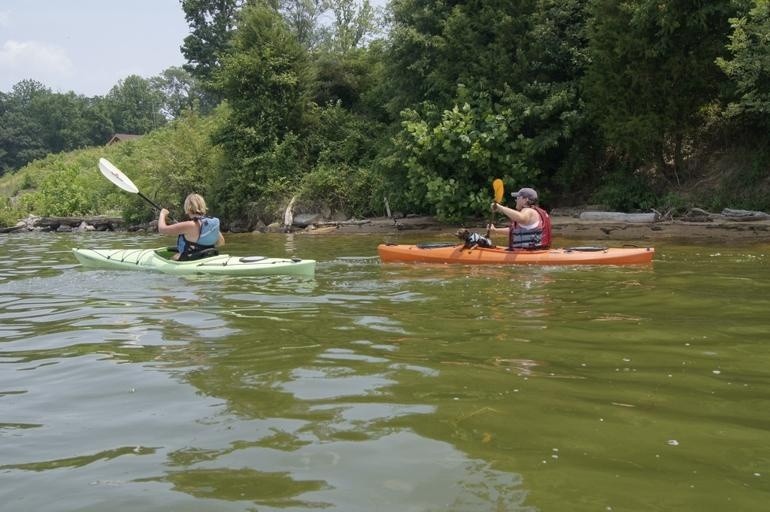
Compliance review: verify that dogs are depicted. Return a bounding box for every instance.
[455,228,493,253]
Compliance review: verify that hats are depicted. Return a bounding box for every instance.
[511,188,537,200]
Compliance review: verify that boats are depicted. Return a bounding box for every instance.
[378,235,659,266]
[68,240,319,276]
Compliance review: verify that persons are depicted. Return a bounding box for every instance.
[485,188,552,251]
[157,193,227,261]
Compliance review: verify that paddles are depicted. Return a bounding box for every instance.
[485,179,504,238]
[98,158,177,223]
